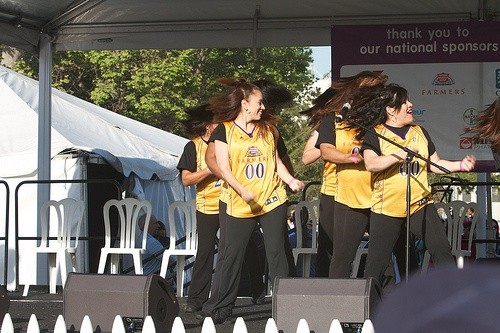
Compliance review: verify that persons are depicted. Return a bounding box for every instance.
[178,77,305,320]
[351,84,476,280]
[302,88,395,295]
[464,97,500,145]
[467,208,474,222]
[136,215,177,288]
[317,72,420,278]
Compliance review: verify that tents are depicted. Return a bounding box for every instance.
[0,65,222,295]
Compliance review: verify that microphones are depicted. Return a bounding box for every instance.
[336,103,351,122]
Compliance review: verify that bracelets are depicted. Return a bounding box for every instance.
[460,161,463,171]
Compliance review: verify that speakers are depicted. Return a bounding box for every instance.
[272,276,382,333]
[374,262,500,333]
[63,272,179,333]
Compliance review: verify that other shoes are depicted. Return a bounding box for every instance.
[195,310,213,325]
[252,287,266,304]
[185,303,202,312]
[213,306,232,324]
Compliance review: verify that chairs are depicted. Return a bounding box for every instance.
[421,200,478,273]
[97,197,152,275]
[159,198,198,298]
[350,247,368,279]
[292,199,320,278]
[21,198,85,298]
